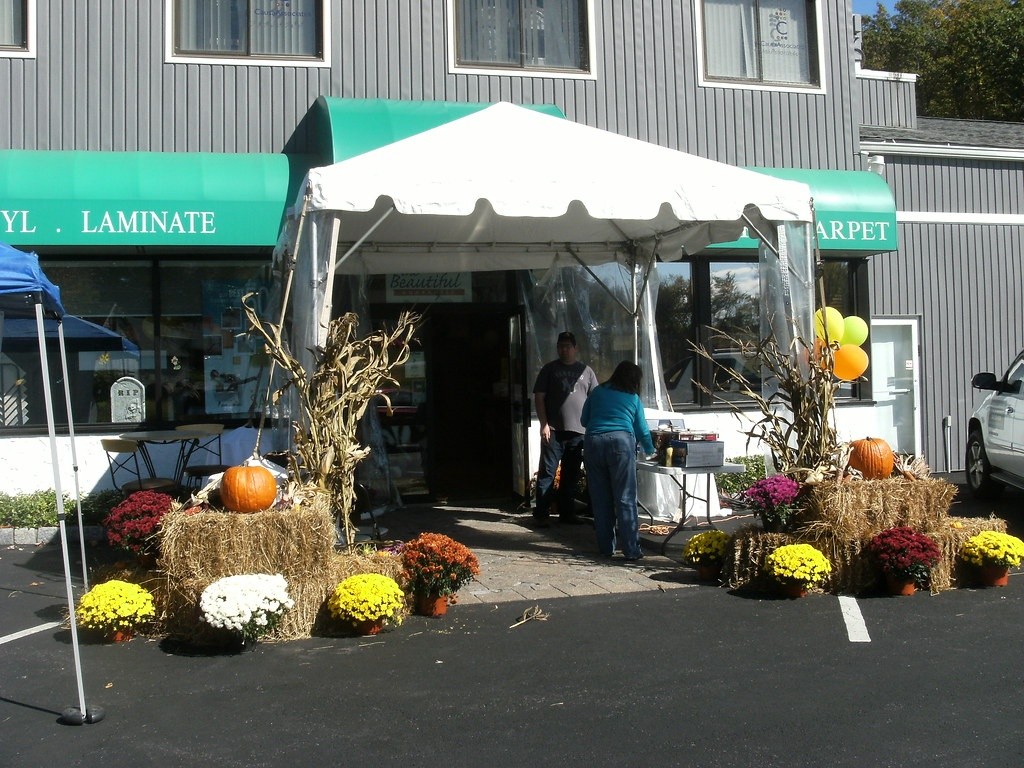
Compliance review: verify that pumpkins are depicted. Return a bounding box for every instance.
[847,436,894,479]
[219,461,277,513]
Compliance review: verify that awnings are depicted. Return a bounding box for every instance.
[0,96,898,252]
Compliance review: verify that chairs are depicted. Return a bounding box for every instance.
[101,439,177,500]
[175,423,231,494]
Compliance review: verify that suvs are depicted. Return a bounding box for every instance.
[965,346,1024,499]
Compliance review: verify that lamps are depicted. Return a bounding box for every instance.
[854,144,886,176]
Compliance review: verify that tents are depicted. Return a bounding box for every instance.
[252,100,816,450]
[0,241,106,725]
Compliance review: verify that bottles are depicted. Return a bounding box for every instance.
[636,441,645,462]
[665,441,673,467]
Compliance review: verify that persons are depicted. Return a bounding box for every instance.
[532,332,598,528]
[145,356,199,416]
[580,360,656,564]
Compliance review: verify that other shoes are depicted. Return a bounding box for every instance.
[626,552,643,560]
[604,553,614,556]
[538,518,550,528]
[559,515,585,524]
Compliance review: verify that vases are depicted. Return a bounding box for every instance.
[102,627,134,643]
[890,574,916,596]
[224,625,258,656]
[350,616,386,637]
[695,556,726,581]
[412,590,451,619]
[139,538,163,565]
[980,564,1011,586]
[781,577,807,599]
[760,507,790,531]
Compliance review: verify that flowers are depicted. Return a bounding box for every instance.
[399,533,479,595]
[684,529,732,564]
[104,489,174,556]
[328,573,402,623]
[199,571,295,636]
[70,581,154,633]
[764,542,832,585]
[957,531,1024,568]
[743,475,798,520]
[872,526,942,579]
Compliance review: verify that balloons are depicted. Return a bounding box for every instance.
[805,307,869,382]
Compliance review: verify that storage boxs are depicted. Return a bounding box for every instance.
[650,428,727,469]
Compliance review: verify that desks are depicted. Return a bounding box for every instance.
[628,462,745,555]
[120,429,203,500]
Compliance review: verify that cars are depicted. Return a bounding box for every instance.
[375,387,424,413]
[659,346,854,406]
